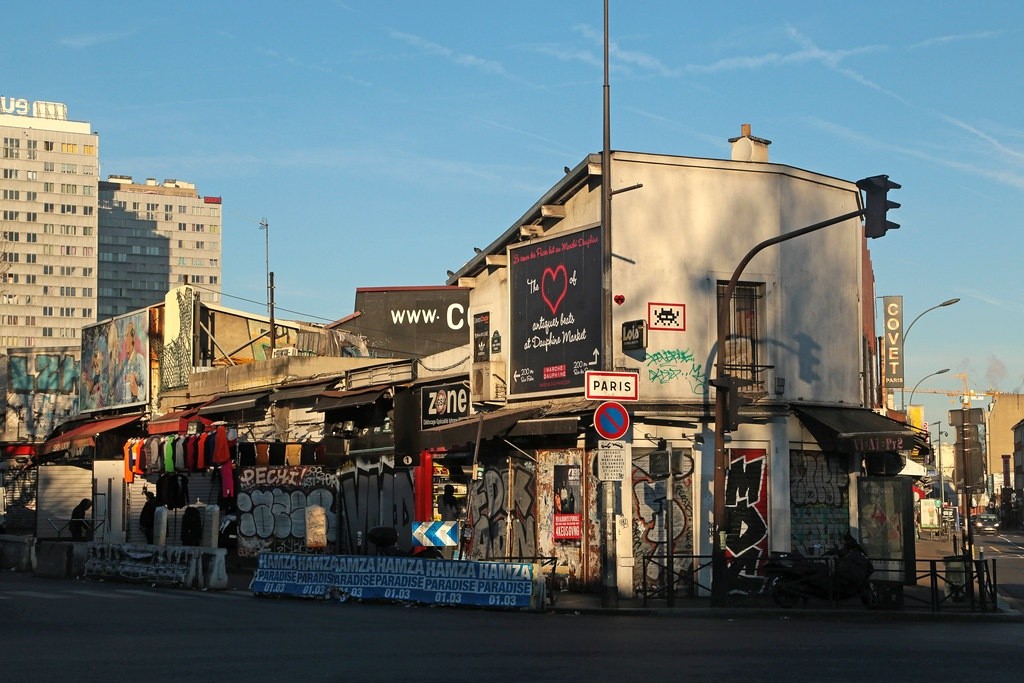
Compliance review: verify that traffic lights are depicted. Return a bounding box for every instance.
[856,173,901,238]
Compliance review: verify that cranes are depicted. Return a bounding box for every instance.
[892,374,999,408]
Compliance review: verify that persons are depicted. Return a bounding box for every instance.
[237,438,256,465]
[555,479,575,515]
[140,492,158,545]
[437,484,461,521]
[268,438,285,466]
[68,498,92,541]
[301,438,318,465]
[284,438,302,467]
[256,437,270,466]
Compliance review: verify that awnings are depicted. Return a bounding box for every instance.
[146,409,191,434]
[305,388,387,413]
[795,405,915,451]
[269,380,337,402]
[197,392,270,415]
[40,415,141,455]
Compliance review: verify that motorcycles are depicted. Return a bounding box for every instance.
[768,527,881,610]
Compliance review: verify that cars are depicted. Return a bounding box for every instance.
[975,517,1002,537]
